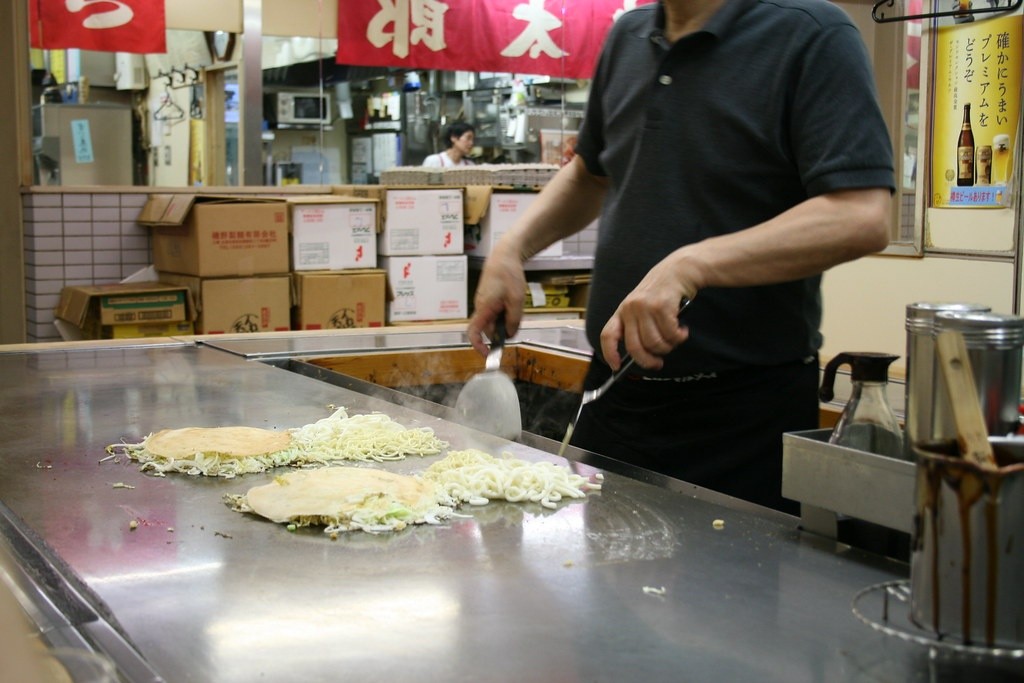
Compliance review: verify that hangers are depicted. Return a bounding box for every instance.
[189,77,203,119]
[151,84,184,119]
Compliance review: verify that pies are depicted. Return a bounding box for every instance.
[247,469,424,523]
[146,427,291,457]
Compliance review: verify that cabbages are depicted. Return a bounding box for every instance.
[223,476,457,536]
[101,435,308,479]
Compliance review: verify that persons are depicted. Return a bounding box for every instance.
[421,121,476,170]
[469,0,896,518]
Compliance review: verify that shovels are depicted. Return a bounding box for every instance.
[557,299,691,456]
[457,304,523,445]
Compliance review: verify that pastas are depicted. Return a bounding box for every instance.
[291,407,449,463]
[428,450,602,512]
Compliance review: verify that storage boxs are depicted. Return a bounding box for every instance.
[522,287,570,308]
[292,270,386,330]
[149,198,289,278]
[100,290,185,325]
[292,196,378,270]
[158,272,296,333]
[333,185,465,256]
[380,258,467,324]
[81,322,193,339]
[468,192,563,257]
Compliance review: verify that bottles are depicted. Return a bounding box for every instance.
[903,300,1024,447]
[956,103,976,186]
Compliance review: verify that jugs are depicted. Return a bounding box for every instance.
[816,351,904,460]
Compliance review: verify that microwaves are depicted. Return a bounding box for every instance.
[265,91,332,128]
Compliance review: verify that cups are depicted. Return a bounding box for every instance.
[992,135,1010,186]
